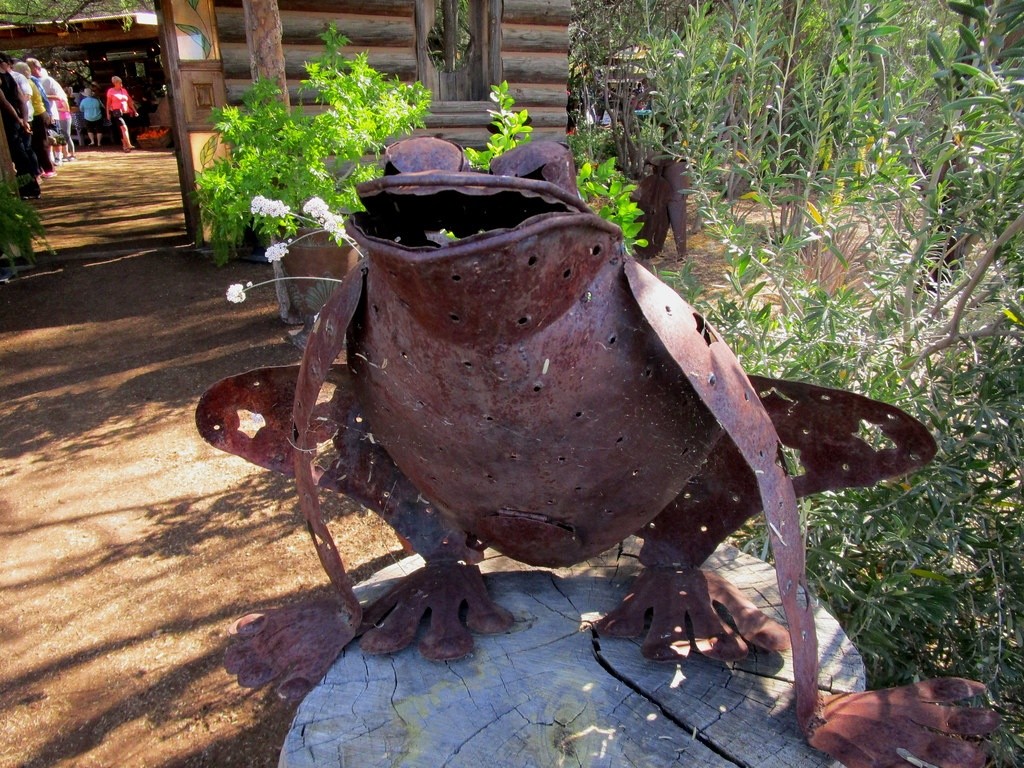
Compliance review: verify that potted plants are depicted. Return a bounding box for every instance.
[191,24,432,323]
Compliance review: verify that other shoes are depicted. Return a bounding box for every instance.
[54,157,62,166]
[43,172,57,178]
[63,156,77,162]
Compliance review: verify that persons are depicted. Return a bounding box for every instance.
[0,52,157,200]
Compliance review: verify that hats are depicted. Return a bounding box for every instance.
[79,88,94,96]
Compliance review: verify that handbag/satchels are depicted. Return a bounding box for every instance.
[112,108,123,118]
